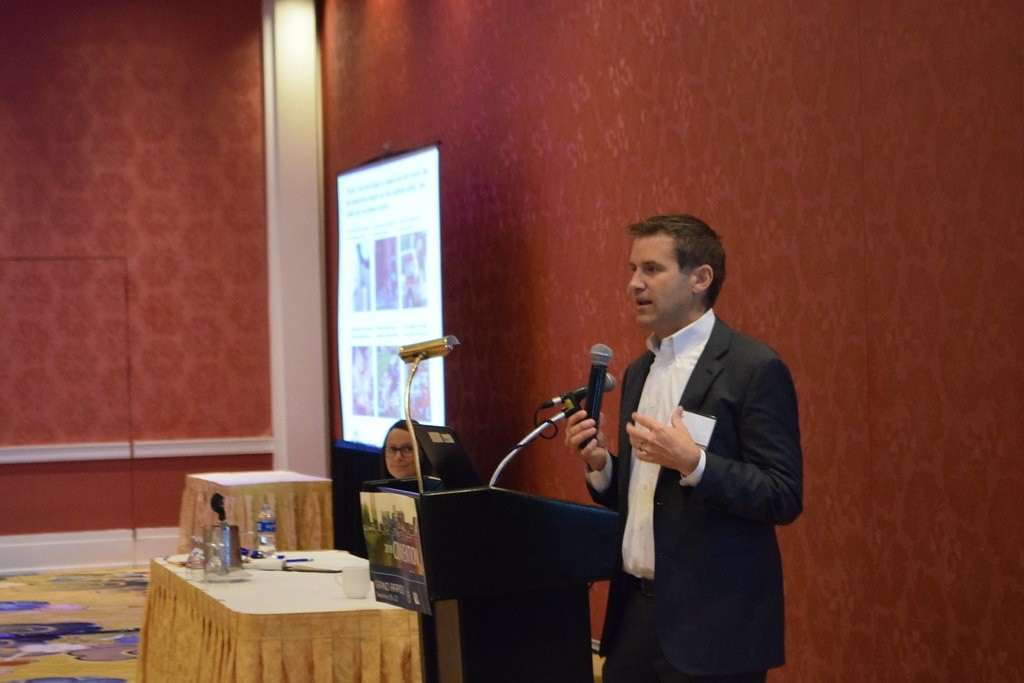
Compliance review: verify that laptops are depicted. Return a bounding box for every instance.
[413,425,484,490]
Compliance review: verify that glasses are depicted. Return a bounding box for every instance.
[382,446,413,457]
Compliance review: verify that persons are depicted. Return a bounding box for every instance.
[565,214,804,683]
[382,421,431,480]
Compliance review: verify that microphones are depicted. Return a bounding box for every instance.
[580,343,614,448]
[539,372,616,410]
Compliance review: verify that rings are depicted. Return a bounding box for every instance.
[639,442,643,451]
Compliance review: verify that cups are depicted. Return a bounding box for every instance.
[335,565,371,599]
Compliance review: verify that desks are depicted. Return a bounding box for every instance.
[135,549,423,683]
[177,469,333,554]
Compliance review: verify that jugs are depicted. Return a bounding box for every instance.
[201,522,258,583]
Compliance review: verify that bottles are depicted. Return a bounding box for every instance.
[256,504,276,559]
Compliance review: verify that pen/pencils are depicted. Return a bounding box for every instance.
[282,558,315,562]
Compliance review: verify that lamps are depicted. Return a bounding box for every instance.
[397,334,461,493]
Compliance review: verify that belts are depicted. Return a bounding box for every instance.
[629,575,657,598]
